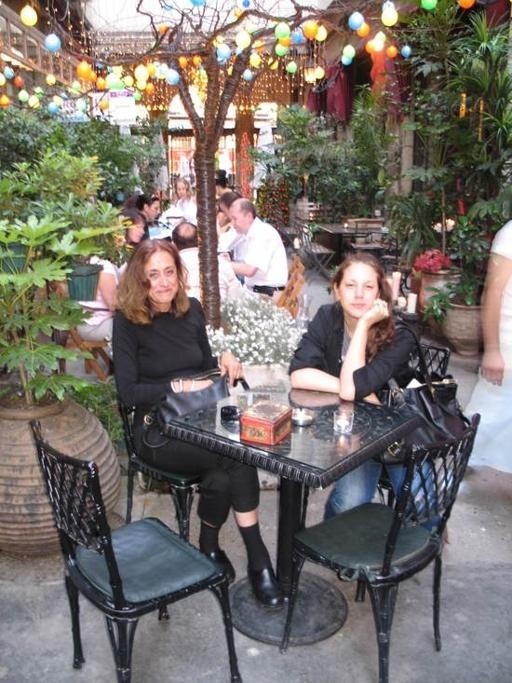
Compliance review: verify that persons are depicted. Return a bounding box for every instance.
[75,167,290,359]
[111,237,285,608]
[288,252,443,534]
[460,219,512,476]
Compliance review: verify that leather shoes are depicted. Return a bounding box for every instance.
[248,567,284,608]
[208,549,235,583]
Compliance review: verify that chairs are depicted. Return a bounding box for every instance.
[29,414,245,682]
[277,412,483,682]
[42,267,113,380]
[290,208,388,282]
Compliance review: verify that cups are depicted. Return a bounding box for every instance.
[333,409,355,434]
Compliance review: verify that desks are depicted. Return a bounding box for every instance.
[147,385,422,645]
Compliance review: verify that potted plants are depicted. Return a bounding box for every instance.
[445,2,509,360]
[0,149,125,558]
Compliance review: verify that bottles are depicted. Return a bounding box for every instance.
[391,271,417,314]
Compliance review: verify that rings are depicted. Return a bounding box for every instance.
[373,301,380,305]
[381,303,386,308]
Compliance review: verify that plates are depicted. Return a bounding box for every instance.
[290,408,315,425]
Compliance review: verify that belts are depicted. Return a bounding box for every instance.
[274,287,285,291]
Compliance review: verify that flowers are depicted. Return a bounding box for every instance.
[414,248,447,274]
[207,284,307,365]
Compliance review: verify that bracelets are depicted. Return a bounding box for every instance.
[191,379,196,391]
[170,380,177,392]
[178,379,184,392]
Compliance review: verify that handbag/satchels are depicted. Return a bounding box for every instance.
[371,320,471,464]
[141,369,250,449]
